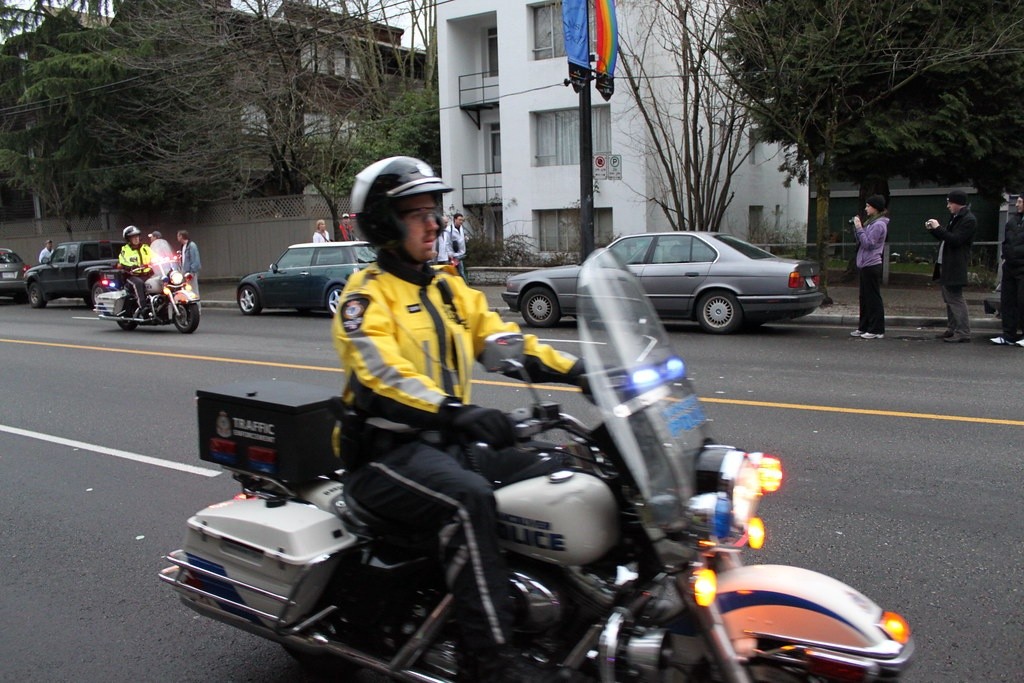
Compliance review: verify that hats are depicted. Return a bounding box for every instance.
[147,231,161,239]
[342,214,350,218]
[865,194,886,212]
[946,190,966,205]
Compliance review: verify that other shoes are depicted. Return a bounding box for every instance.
[142,307,149,319]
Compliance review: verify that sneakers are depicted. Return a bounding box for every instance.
[1016,339,1024,346]
[935,330,954,339]
[944,334,970,343]
[861,332,884,339]
[850,330,865,336]
[458,642,582,683]
[990,337,1014,345]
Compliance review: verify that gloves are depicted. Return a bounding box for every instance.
[439,397,532,451]
[567,357,624,405]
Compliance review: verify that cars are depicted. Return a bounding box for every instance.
[235,239,377,317]
[502,230,825,335]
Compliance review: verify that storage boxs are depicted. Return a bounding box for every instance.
[178,492,358,632]
[195,379,343,482]
[84,265,113,289]
[95,291,128,316]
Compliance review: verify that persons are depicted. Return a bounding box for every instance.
[312,219,330,243]
[450,213,470,287]
[925,190,977,343]
[332,155,619,683]
[337,213,359,242]
[177,230,201,317]
[849,194,890,339]
[427,216,452,265]
[118,225,163,320]
[38,240,54,264]
[148,231,168,258]
[989,190,1024,347]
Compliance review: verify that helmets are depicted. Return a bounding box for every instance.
[123,226,142,240]
[350,157,455,244]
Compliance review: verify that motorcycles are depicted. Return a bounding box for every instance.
[160,246,912,683]
[93,242,202,334]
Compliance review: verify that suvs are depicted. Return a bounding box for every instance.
[0,248,30,303]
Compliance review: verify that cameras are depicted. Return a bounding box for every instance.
[927,222,931,226]
[849,218,855,225]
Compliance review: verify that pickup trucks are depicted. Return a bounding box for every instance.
[22,239,121,310]
